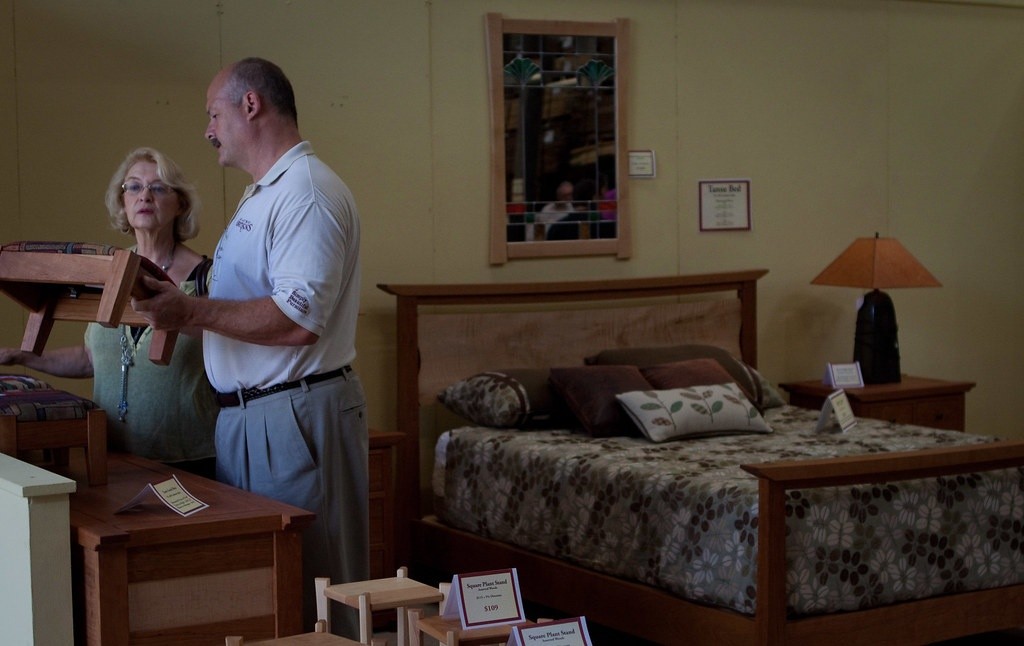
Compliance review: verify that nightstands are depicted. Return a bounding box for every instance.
[777,372,976,433]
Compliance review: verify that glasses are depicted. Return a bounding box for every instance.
[122,181,174,196]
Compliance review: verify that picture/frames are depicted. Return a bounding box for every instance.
[484,10,634,267]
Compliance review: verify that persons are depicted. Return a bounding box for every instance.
[0,147,215,477]
[132,57,369,641]
[534,177,617,239]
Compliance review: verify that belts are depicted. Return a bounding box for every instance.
[215,364,352,408]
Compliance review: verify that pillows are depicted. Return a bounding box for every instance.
[615,381,774,442]
[436,367,578,428]
[640,358,765,417]
[584,344,787,408]
[549,364,654,439]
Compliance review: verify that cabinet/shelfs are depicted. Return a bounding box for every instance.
[407,582,555,646]
[224,620,372,646]
[0,445,318,646]
[368,427,408,633]
[314,565,445,646]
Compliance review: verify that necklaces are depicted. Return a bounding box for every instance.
[118,242,176,422]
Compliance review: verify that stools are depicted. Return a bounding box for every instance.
[0,241,182,367]
[0,387,109,488]
[0,373,55,463]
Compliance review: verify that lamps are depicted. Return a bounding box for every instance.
[808,229,944,386]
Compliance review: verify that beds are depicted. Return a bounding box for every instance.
[372,267,1024,646]
